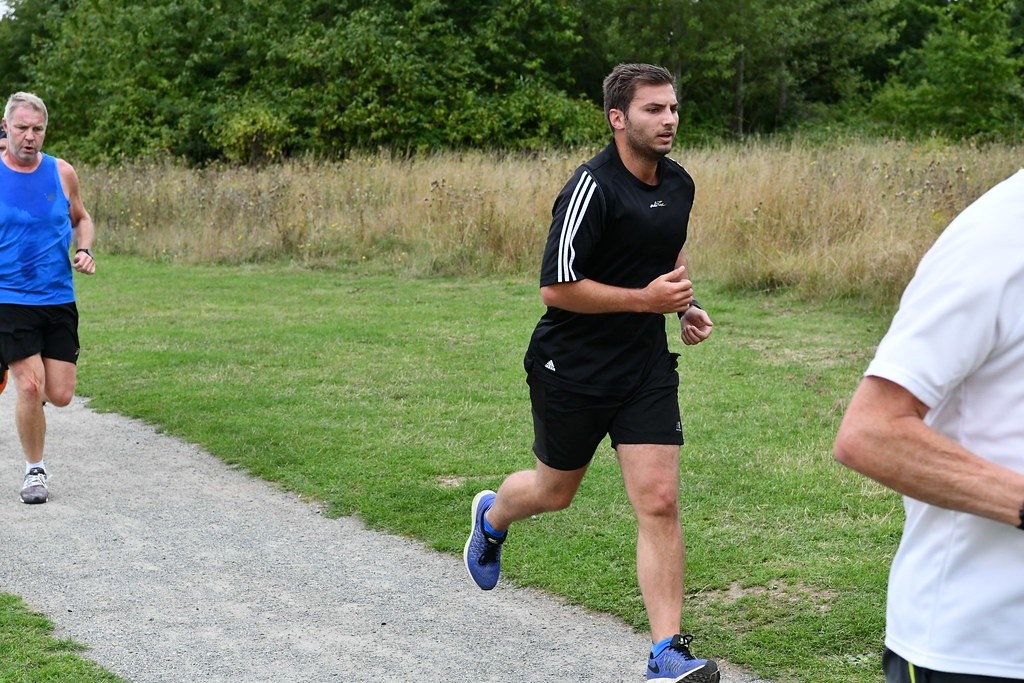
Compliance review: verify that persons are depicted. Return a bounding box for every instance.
[463,66,719,683]
[0,90,95,503]
[833,170,1024,683]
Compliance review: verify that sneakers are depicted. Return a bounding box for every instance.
[20,466,48,503]
[462,490,508,591]
[647,634,721,683]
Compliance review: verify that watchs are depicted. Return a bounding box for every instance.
[75,249,94,260]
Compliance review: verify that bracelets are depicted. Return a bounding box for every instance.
[678,300,701,318]
[1018,503,1024,529]
